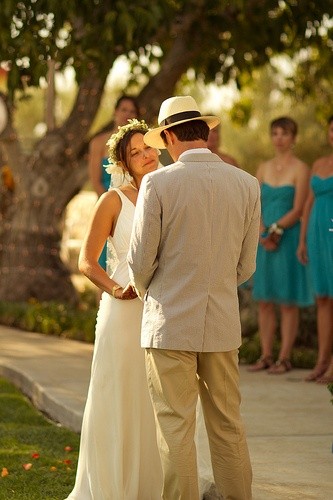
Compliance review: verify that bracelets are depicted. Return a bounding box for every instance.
[270,222,283,235]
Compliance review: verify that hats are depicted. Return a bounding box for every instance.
[143,96,221,149]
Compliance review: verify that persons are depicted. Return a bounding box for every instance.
[126,95,261,500]
[89,94,333,384]
[62,119,215,500]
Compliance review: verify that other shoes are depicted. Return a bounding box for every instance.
[305,360,333,383]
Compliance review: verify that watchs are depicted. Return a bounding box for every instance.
[112,285,122,297]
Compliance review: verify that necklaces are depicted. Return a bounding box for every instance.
[129,181,138,191]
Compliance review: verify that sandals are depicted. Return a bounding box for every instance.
[246,355,273,371]
[268,359,293,374]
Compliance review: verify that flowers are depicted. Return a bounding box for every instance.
[103,118,148,190]
